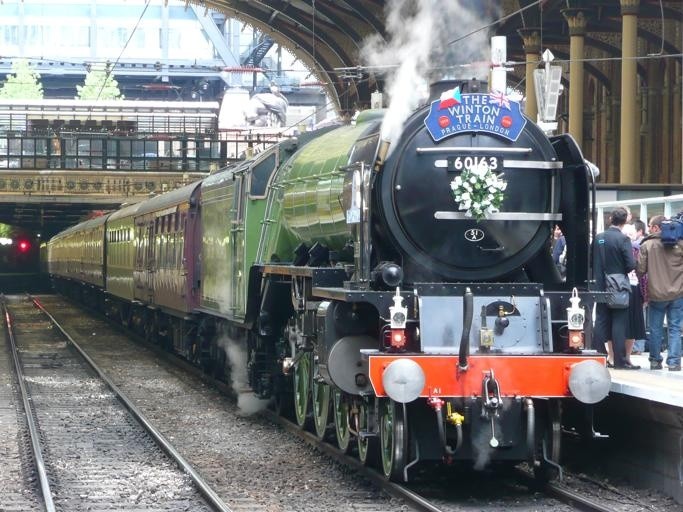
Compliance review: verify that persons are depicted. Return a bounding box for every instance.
[551,206,683,371]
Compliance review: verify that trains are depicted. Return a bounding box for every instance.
[40,78,612,483]
[0,56,299,100]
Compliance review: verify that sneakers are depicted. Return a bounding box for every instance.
[607,351,680,371]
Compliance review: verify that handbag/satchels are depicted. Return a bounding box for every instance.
[602,272,631,310]
